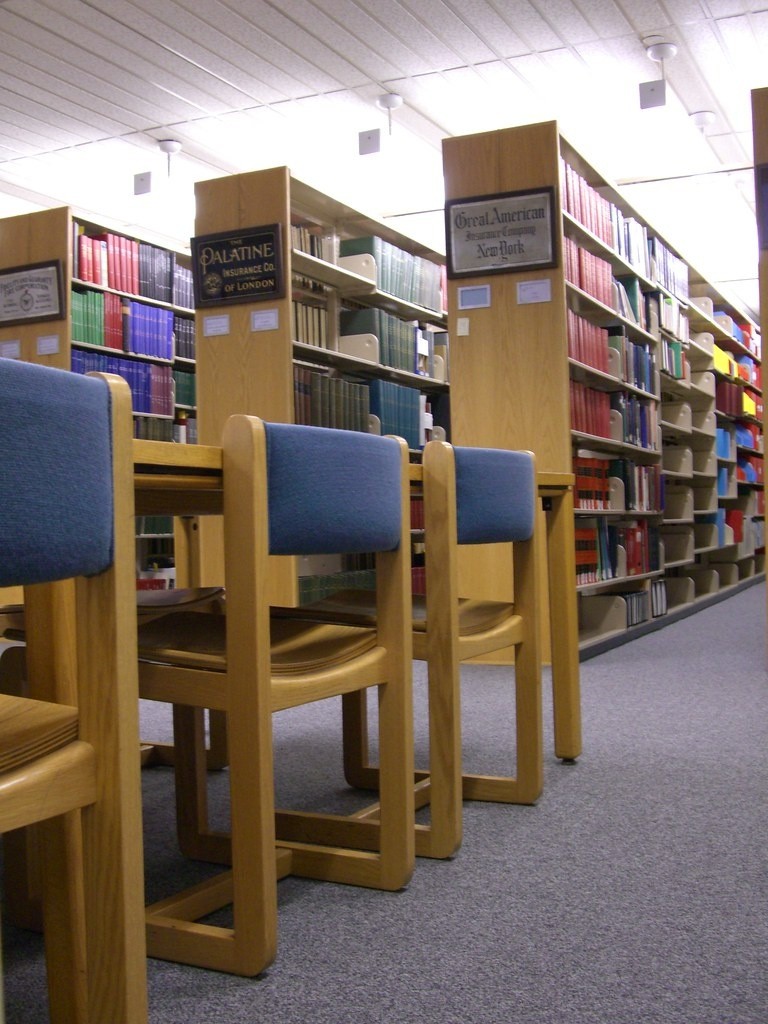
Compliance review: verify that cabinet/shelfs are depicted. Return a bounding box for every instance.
[191,166,447,608]
[437,117,768,667]
[0,204,194,604]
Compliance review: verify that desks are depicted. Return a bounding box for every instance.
[132,435,583,765]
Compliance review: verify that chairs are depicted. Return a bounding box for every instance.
[271,439,544,861]
[0,357,150,1024]
[137,414,416,979]
[0,586,230,771]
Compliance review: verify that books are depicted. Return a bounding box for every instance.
[290,223,452,598]
[71,221,197,589]
[559,157,766,626]
